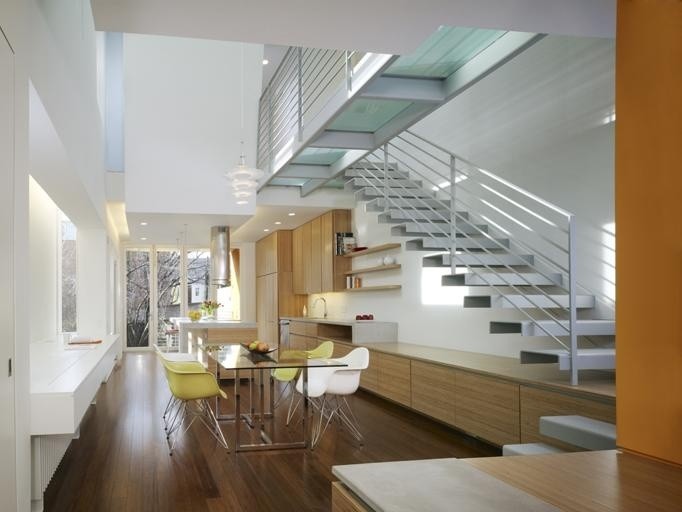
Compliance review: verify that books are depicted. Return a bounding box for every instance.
[336,233,367,256]
[345,276,361,290]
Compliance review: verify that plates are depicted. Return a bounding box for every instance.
[240,344,276,354]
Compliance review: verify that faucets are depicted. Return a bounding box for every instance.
[312,297,327,318]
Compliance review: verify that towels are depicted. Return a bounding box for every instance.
[68,338,102,344]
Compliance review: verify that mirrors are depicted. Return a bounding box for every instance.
[29,171,86,383]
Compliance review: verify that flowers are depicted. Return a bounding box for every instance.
[199,300,224,315]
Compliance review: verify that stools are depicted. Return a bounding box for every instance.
[165,329,180,351]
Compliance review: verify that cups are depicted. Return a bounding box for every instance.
[356,315,374,319]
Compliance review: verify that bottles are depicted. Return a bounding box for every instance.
[342,232,356,254]
[347,276,362,288]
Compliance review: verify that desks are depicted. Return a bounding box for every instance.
[169,316,241,353]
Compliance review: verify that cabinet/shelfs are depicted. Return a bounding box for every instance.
[331,479,374,512]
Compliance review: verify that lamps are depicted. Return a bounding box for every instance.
[224,42,257,206]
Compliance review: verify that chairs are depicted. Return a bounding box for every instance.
[152,340,228,456]
